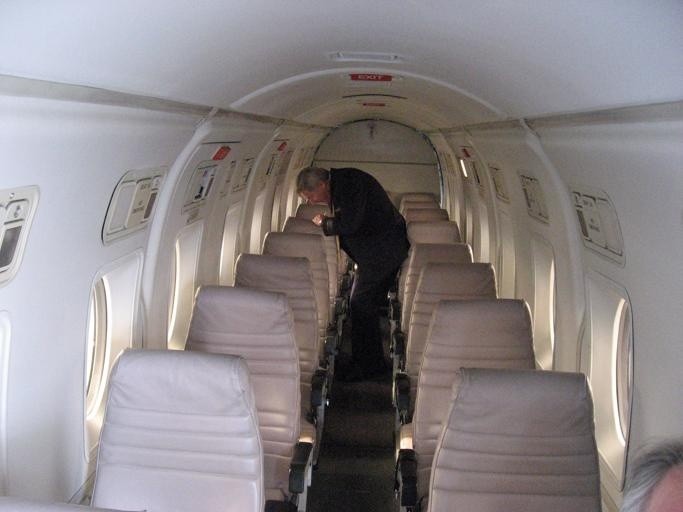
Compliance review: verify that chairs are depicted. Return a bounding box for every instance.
[392,296,537,512]
[389,261,500,436]
[183,283,317,512]
[388,193,476,360]
[90,344,289,512]
[426,362,603,511]
[230,251,331,431]
[261,197,357,352]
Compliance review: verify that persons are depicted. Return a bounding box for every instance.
[296,167,410,383]
[620,435,683,512]
